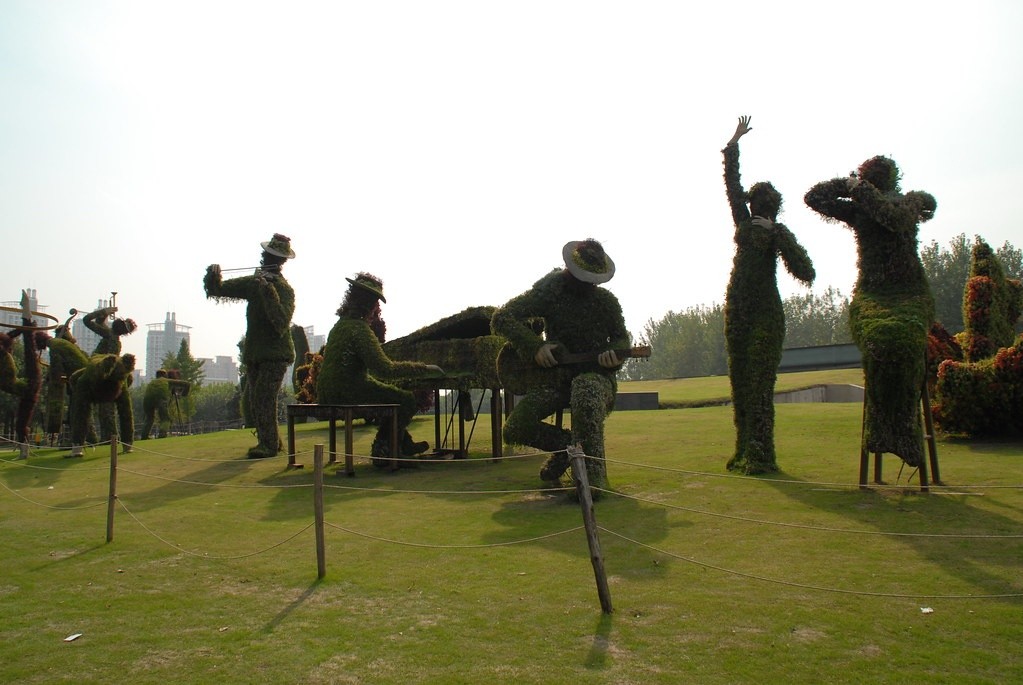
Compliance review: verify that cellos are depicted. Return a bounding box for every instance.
[44,307,78,433]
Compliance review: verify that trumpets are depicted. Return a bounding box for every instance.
[109,291,118,320]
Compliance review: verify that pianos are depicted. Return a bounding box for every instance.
[368,304,517,463]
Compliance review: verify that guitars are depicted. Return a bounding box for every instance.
[496,337,652,397]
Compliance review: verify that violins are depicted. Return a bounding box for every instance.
[254,269,280,284]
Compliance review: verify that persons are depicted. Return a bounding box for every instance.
[491,240,631,503]
[203,233,296,459]
[316,274,448,466]
[804,154,938,466]
[139,369,191,441]
[721,117,816,478]
[0,289,137,461]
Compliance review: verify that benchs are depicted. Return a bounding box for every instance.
[286,403,401,477]
